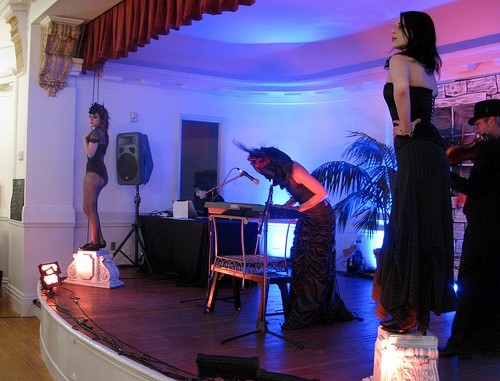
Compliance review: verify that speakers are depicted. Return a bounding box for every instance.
[195,352,260,381]
[116,132,153,185]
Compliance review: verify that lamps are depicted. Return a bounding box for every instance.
[336,245,357,263]
[38,261,61,277]
[41,273,63,294]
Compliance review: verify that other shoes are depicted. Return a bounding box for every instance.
[76,243,100,251]
[437,345,472,361]
[99,240,106,248]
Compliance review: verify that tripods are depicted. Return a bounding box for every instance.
[179,175,239,304]
[110,186,153,275]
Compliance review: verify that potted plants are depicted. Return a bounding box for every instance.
[309,130,398,267]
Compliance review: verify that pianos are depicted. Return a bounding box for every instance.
[204,200,308,219]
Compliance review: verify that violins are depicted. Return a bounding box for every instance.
[445,131,489,166]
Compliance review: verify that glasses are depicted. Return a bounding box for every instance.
[473,118,496,129]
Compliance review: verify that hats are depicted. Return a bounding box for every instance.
[469,99,500,125]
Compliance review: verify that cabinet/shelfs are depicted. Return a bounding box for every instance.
[206,214,299,330]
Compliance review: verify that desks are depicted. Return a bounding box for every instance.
[137,215,259,289]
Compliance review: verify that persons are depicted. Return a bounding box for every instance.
[378,10,460,335]
[247,146,355,331]
[441,98,500,363]
[194,180,226,217]
[78,102,109,249]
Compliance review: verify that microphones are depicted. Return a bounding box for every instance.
[236,168,259,185]
[268,178,273,206]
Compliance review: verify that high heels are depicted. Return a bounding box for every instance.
[379,309,429,336]
